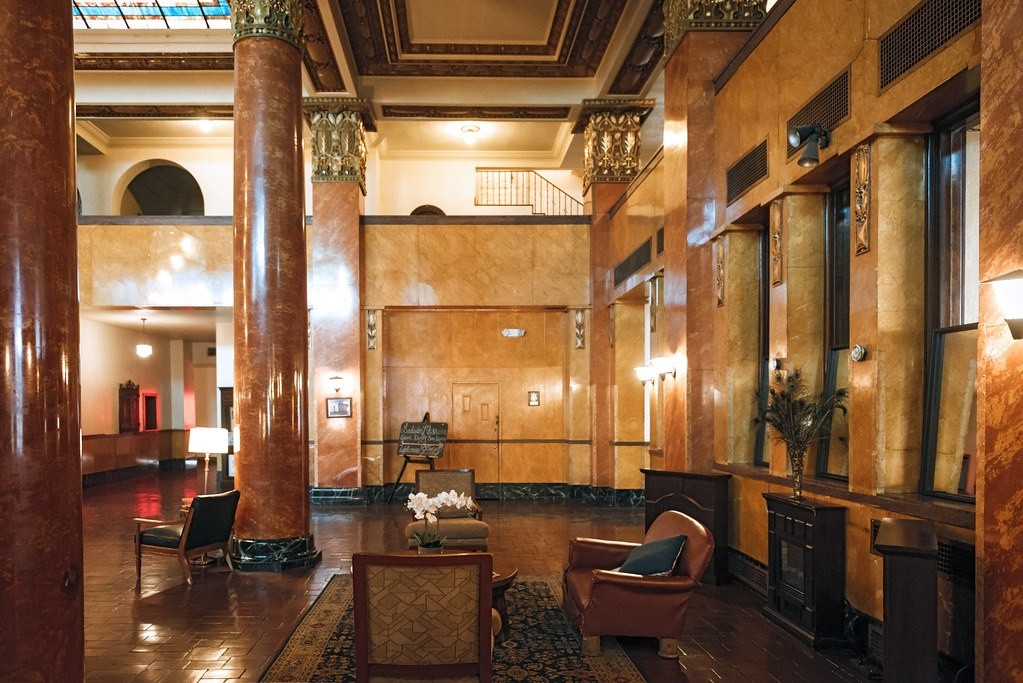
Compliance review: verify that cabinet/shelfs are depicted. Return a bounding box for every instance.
[873,517,939,683]
[762,492,845,649]
[640,467,733,586]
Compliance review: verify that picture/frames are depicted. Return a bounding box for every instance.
[326,398,352,418]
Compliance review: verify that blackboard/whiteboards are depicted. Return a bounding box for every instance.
[396,420,447,459]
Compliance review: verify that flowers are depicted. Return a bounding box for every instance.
[407,489,477,546]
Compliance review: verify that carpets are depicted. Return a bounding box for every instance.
[255,573,650,682]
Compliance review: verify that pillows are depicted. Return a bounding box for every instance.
[619,535,687,576]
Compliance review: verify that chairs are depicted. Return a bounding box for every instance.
[132,490,241,586]
[563,509,715,657]
[406,469,489,552]
[353,553,501,683]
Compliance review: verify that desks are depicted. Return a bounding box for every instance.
[493,559,518,642]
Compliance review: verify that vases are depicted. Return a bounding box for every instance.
[418,546,443,555]
[787,444,806,500]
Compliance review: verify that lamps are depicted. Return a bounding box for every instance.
[329,376,343,392]
[981,269,1023,340]
[788,121,831,168]
[184,427,229,564]
